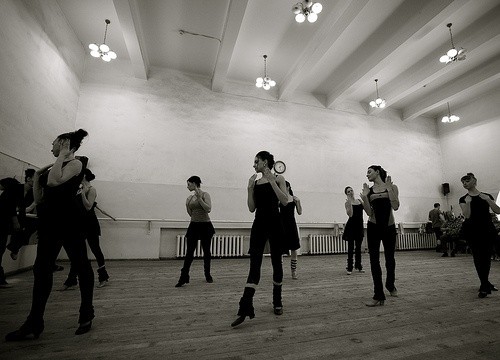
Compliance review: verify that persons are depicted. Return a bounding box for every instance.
[0,167,37,261]
[230,151,288,328]
[279,181,302,279]
[4,129,95,340]
[362,165,400,307]
[53,168,116,290]
[342,187,364,274]
[429,203,455,256]
[457,173,500,298]
[175,176,216,287]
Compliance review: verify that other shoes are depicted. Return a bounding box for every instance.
[451,253,455,257]
[7,243,20,260]
[292,272,298,279]
[491,286,498,291]
[442,253,448,256]
[56,266,64,271]
[479,291,487,298]
[0,284,13,288]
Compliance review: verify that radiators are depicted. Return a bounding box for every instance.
[309,233,354,254]
[394,232,438,250]
[175,235,244,257]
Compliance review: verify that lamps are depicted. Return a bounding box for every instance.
[368,79,386,109]
[89,18,118,62]
[255,54,277,91]
[439,22,466,64]
[291,0,322,24]
[441,101,459,123]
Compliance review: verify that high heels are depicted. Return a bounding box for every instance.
[347,268,352,275]
[274,307,283,314]
[355,265,362,270]
[231,313,254,327]
[56,284,77,290]
[6,328,44,341]
[97,279,108,287]
[390,288,398,296]
[206,275,213,282]
[75,321,92,335]
[175,279,189,287]
[365,298,384,306]
